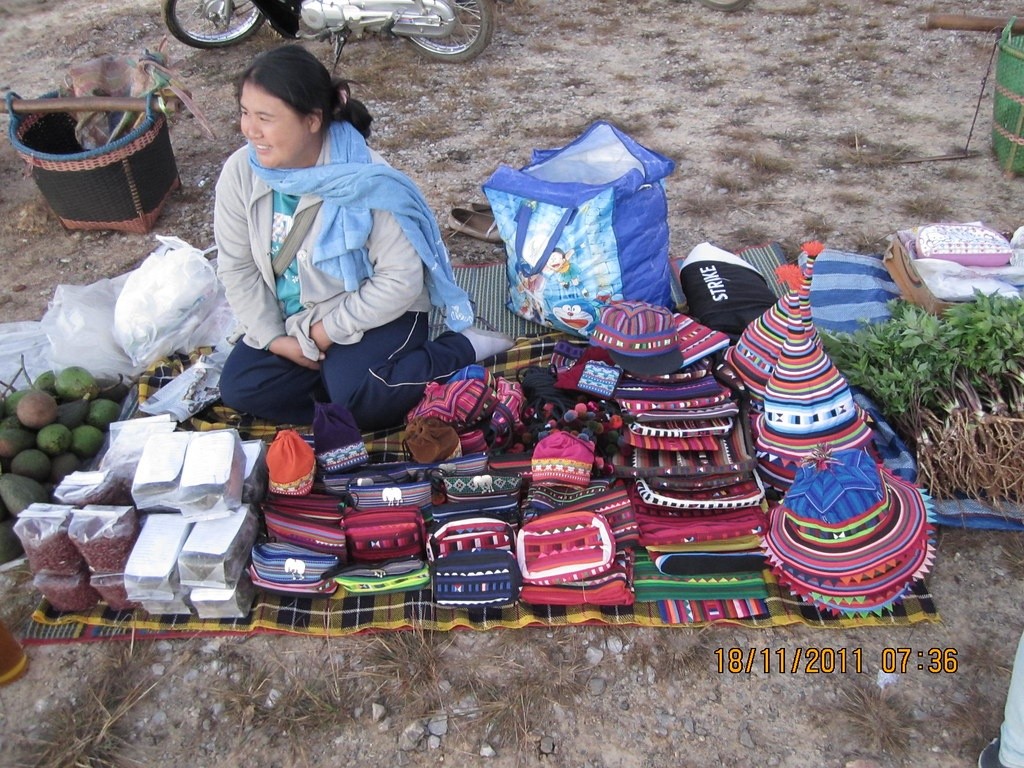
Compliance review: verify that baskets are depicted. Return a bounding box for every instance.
[991,16,1024,172]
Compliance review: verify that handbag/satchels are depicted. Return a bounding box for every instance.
[481,121,675,333]
[110,234,219,369]
[248,312,770,605]
[6,90,181,233]
[913,224,1013,268]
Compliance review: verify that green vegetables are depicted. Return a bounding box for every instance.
[819,286,1024,517]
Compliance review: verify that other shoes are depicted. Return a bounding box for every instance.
[447,201,503,242]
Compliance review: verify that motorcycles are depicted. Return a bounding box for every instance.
[162,0,496,81]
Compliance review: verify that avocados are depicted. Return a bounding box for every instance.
[0,368,129,566]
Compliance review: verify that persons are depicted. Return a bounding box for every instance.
[212,43,515,426]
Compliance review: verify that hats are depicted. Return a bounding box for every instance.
[719,239,939,621]
[588,301,683,375]
